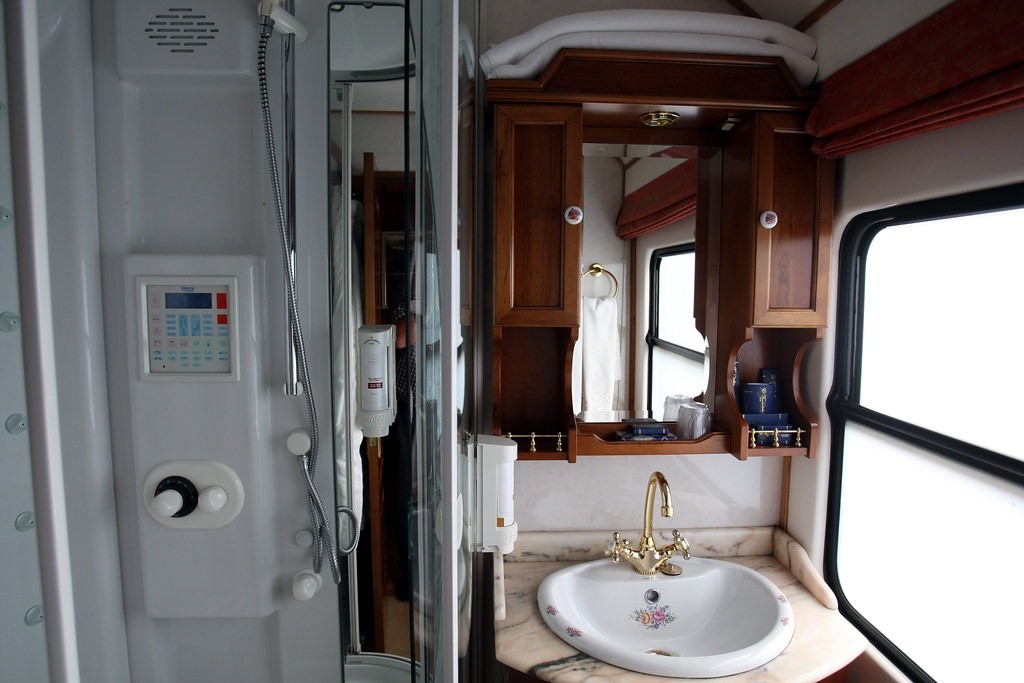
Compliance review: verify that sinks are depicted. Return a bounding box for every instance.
[536,554,795,677]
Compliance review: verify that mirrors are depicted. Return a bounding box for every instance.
[571,142,724,422]
[328,0,424,665]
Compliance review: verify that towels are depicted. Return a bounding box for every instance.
[582,297,622,411]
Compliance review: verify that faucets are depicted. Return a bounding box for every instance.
[602,470,689,573]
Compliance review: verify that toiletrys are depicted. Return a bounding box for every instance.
[476,436,518,557]
[354,323,397,440]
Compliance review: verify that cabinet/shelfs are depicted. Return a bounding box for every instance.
[484,47,838,462]
